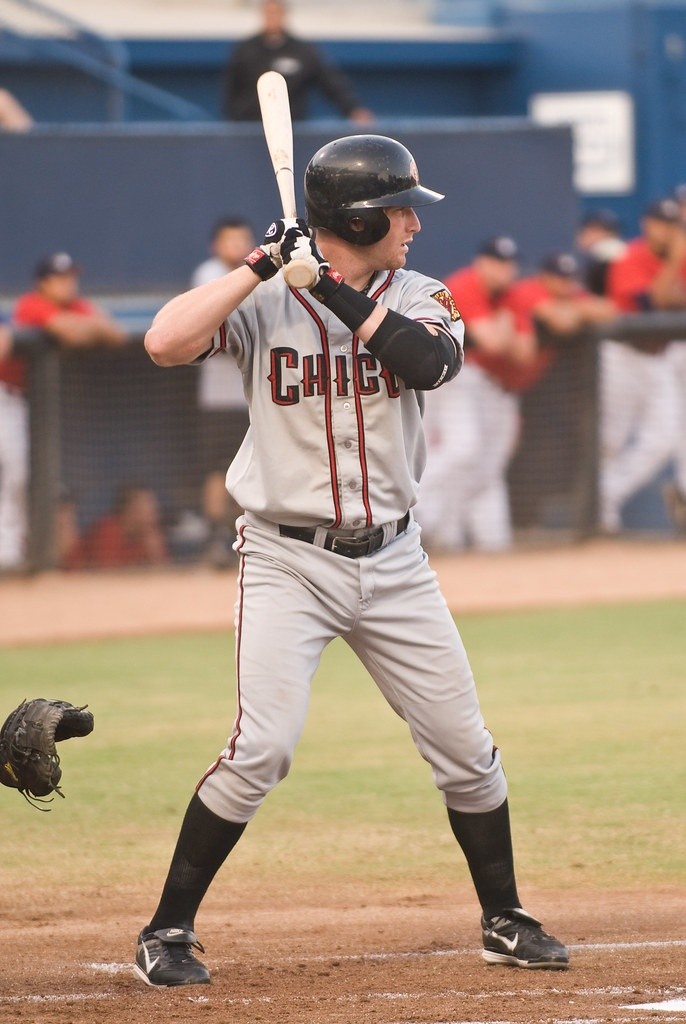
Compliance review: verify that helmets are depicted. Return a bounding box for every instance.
[303,134,445,245]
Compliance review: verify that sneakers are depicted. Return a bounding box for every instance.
[132,924,210,988]
[481,909,570,969]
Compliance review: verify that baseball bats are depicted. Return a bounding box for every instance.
[256,70,316,289]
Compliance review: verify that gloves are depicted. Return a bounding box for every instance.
[243,216,313,281]
[278,228,345,303]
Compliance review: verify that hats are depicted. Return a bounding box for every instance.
[647,199,680,223]
[479,237,517,261]
[596,211,620,231]
[34,254,80,274]
[542,250,581,276]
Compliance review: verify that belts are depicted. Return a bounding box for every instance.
[279,512,410,559]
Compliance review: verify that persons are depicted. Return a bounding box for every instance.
[222,0,376,124]
[134,134,572,988]
[182,185,686,552]
[0,252,126,578]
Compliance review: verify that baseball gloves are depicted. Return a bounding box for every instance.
[1,697,95,813]
